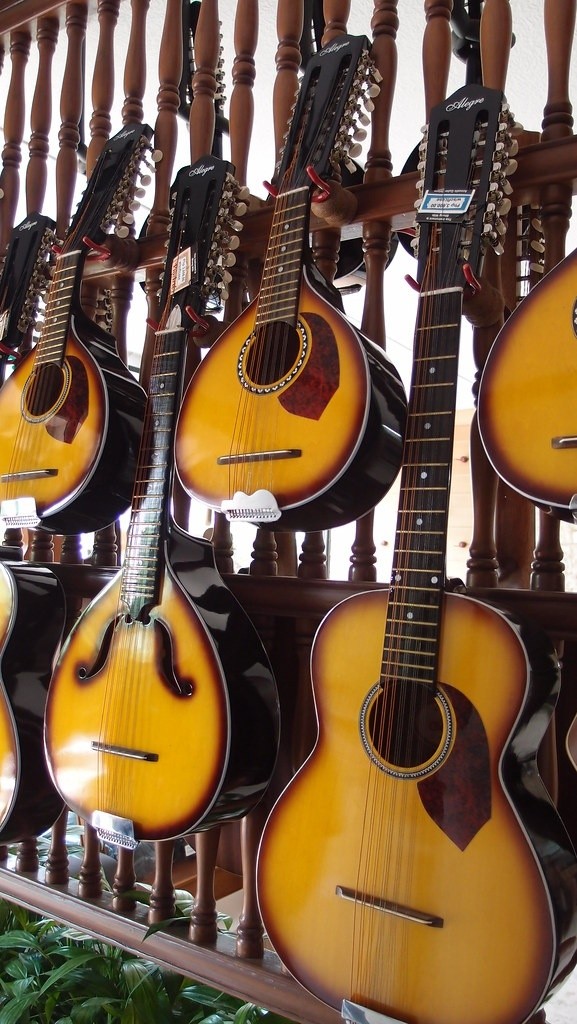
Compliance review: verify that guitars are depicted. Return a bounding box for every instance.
[477,249,577,524]
[42,154,283,843]
[252,83,577,1024]
[0,121,163,536]
[0,213,68,845]
[173,31,409,533]
